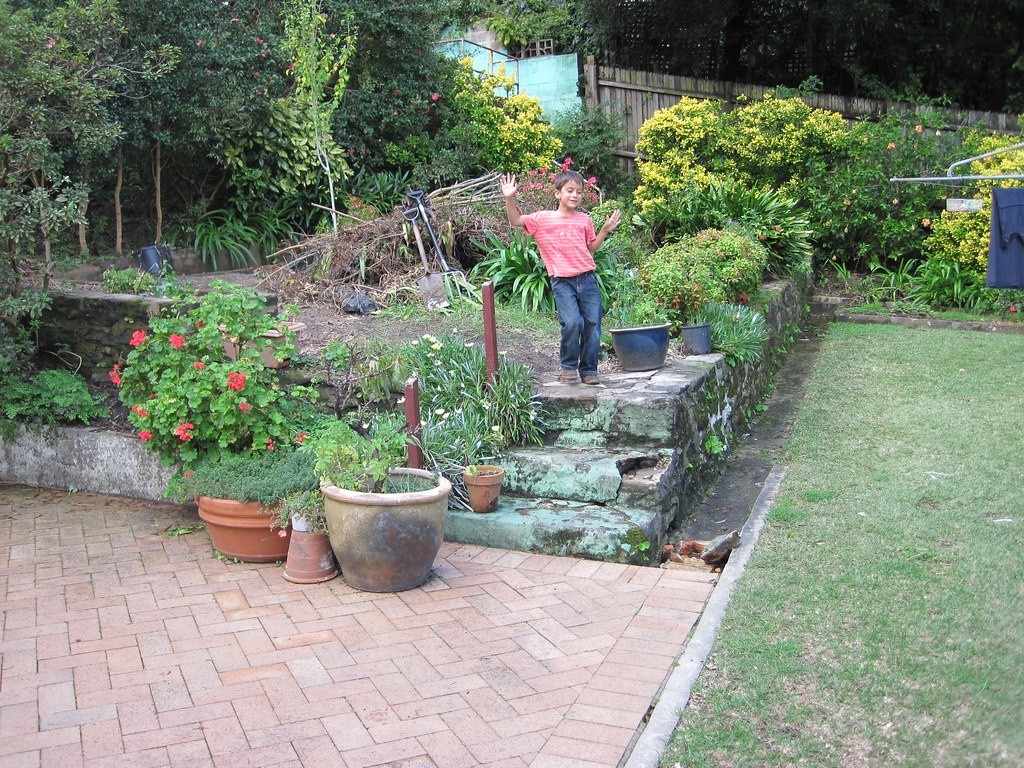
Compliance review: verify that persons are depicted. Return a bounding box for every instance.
[500,171,622,386]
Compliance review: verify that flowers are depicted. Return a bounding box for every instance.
[266,493,331,537]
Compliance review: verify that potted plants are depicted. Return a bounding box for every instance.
[189,454,318,562]
[463,426,505,513]
[131,240,174,276]
[305,419,452,593]
[679,303,712,354]
[607,263,674,372]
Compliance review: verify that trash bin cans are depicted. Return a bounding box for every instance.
[133,244,173,276]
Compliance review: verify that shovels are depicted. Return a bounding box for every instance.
[404,208,451,310]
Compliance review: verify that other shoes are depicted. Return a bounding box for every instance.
[557,366,578,382]
[581,375,600,384]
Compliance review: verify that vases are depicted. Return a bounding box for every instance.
[293,512,313,532]
[199,318,308,368]
[283,530,340,584]
